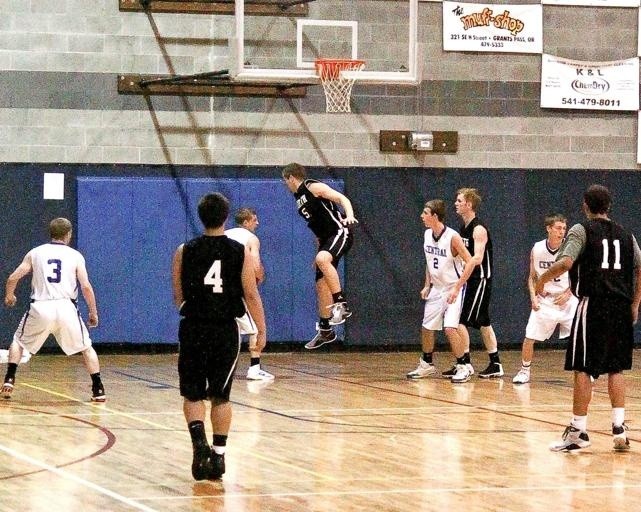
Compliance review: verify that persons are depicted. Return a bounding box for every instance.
[171,192,267,480]
[281,163,358,350]
[224,380,277,511]
[413,376,632,511]
[534,183,640,453]
[1,217,106,403]
[512,213,581,383]
[224,208,276,381]
[442,188,504,378]
[406,199,478,384]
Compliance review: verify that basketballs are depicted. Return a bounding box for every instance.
[256,264,264,284]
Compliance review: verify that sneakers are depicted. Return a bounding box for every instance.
[328,301,352,325]
[208,454,225,478]
[304,328,337,349]
[192,440,215,479]
[0,376,14,398]
[247,363,275,380]
[409,362,532,385]
[550,426,591,452]
[612,423,629,449]
[91,384,105,402]
